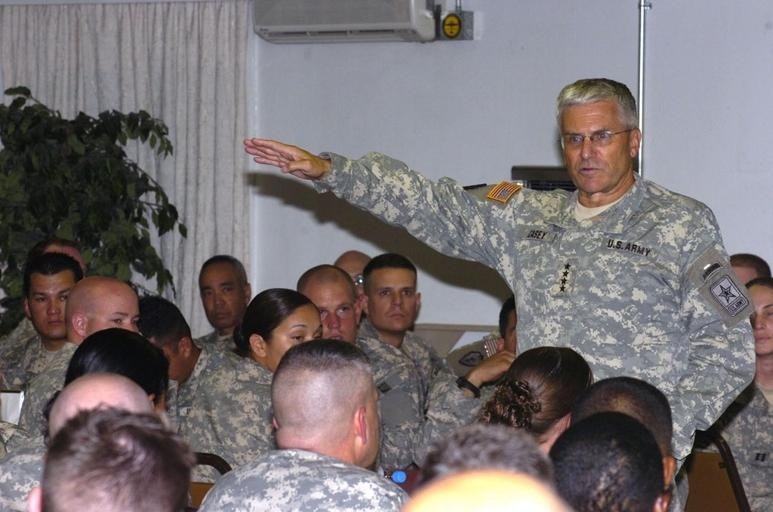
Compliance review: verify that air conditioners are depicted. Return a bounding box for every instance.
[249,1,450,42]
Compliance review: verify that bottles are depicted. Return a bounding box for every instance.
[384,470,407,484]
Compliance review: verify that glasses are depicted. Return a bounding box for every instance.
[561,128,632,142]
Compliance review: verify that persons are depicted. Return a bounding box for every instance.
[695,253,773,512]
[243,77,759,512]
[2,239,676,512]
